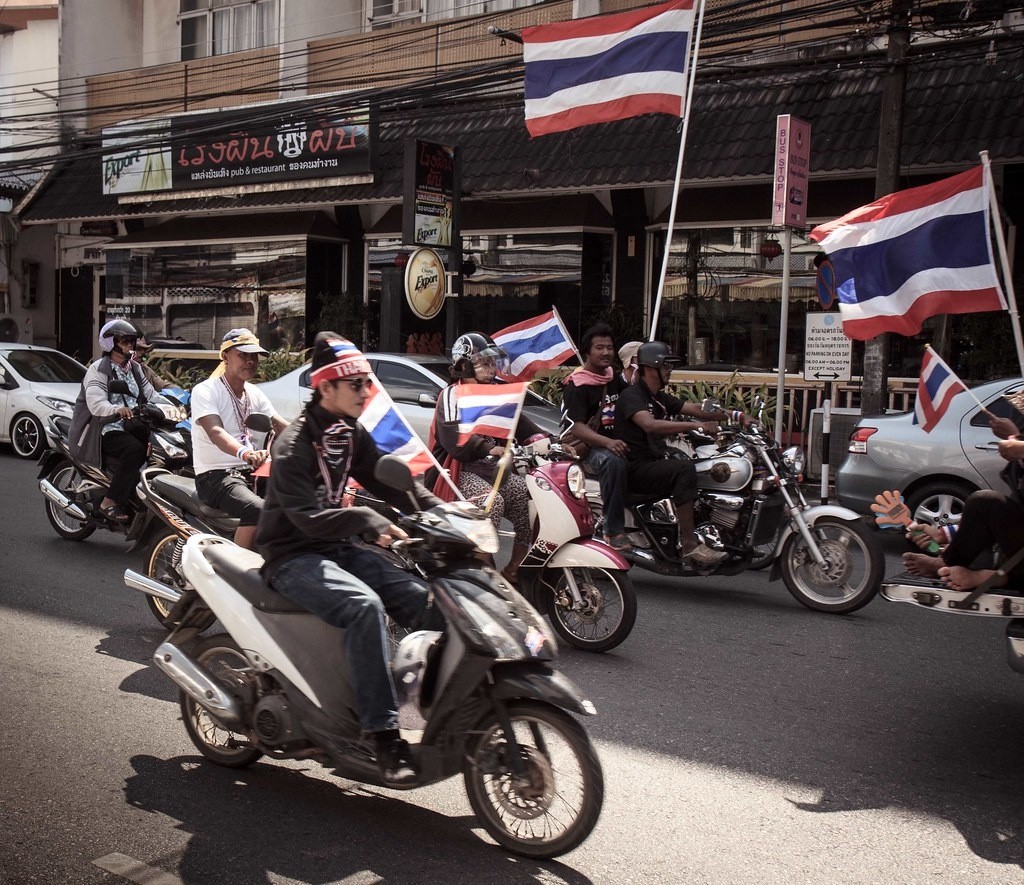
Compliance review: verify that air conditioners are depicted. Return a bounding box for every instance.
[807,407,903,481]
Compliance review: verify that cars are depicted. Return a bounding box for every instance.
[0,343,89,460]
[252,353,568,459]
[836,378,1023,553]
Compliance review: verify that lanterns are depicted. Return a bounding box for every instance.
[760,240,782,263]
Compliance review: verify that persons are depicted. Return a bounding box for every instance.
[189,327,291,553]
[69,320,176,523]
[422,324,1023,596]
[249,333,448,788]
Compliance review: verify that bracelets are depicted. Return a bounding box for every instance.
[234,444,250,461]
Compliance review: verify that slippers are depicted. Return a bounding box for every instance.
[100,504,130,523]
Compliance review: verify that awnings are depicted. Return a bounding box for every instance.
[659,272,821,304]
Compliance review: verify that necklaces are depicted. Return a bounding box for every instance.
[221,374,253,435]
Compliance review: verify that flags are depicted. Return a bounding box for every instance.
[455,381,530,446]
[912,351,965,433]
[809,166,1010,341]
[357,372,436,479]
[521,1,699,138]
[488,311,578,385]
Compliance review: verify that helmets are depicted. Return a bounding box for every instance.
[638,342,681,368]
[98,320,137,353]
[451,332,506,368]
[391,628,446,720]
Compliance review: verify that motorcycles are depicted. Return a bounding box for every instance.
[110,455,604,860]
[520,431,641,651]
[566,398,887,616]
[130,463,293,630]
[27,383,240,543]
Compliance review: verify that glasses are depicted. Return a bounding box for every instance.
[664,363,673,369]
[332,378,372,392]
[117,338,135,346]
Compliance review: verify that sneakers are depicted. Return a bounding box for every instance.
[680,545,730,568]
[376,737,418,787]
[753,547,765,559]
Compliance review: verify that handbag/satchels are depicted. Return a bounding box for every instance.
[556,377,608,462]
[662,433,699,458]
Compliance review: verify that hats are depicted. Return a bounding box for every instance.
[222,328,271,358]
[136,336,155,352]
[619,341,643,368]
[309,331,372,389]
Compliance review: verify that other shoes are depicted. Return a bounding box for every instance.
[602,533,631,551]
